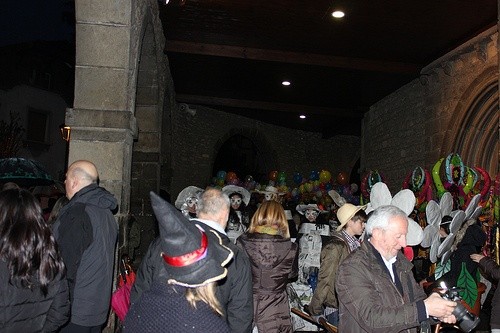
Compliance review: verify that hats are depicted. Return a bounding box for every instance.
[149,192,234,287]
[296,203,321,215]
[222,185,251,207]
[259,185,286,195]
[336,203,367,231]
[328,189,347,207]
[175,186,205,217]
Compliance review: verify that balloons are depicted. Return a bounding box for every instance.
[212,170,358,213]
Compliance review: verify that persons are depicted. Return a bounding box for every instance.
[48,182,500,333]
[53,161,116,333]
[0,180,67,333]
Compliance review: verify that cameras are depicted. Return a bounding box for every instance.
[432,287,480,332]
